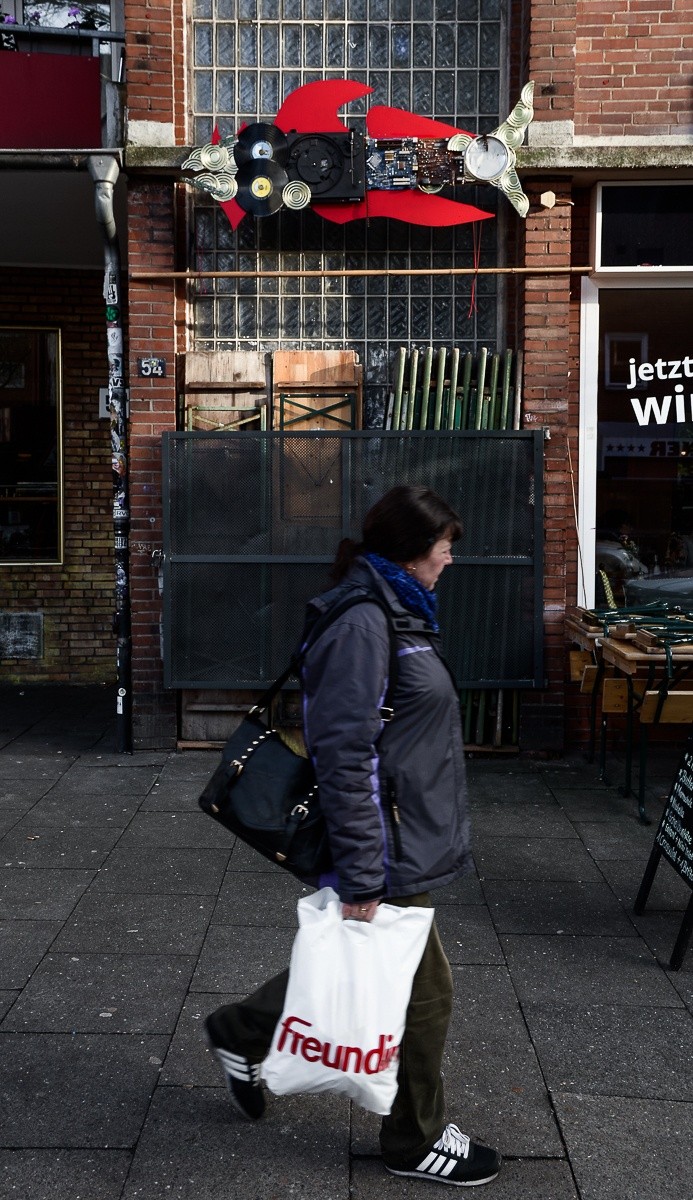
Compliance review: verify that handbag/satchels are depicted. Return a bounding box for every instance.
[259,891,435,1116]
[199,597,397,889]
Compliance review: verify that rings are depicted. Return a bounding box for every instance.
[360,908,368,911]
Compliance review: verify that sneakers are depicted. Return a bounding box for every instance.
[382,1118,501,1185]
[202,1014,268,1118]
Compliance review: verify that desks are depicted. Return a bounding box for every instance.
[597,636,693,799]
[564,617,693,769]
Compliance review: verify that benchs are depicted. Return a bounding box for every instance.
[564,609,693,826]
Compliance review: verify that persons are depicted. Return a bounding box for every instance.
[200,486,502,1185]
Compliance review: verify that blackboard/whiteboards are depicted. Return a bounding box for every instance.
[653,734,693,887]
